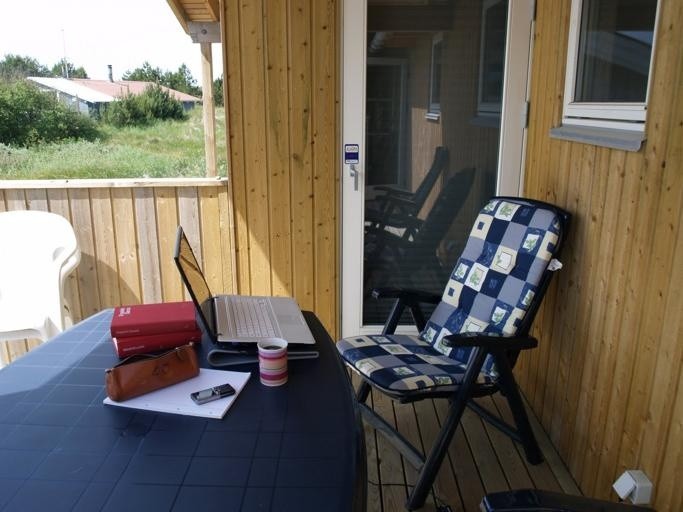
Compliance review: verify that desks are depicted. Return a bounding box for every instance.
[0,306,367,511]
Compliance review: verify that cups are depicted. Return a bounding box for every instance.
[256,337,288,387]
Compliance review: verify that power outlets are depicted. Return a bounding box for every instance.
[613,469,651,503]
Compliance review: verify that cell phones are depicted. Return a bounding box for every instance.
[190,383,236,406]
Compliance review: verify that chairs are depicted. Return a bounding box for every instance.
[336,195,581,507]
[1,209,82,367]
[363,171,479,325]
[365,146,450,241]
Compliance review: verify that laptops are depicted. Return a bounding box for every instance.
[174,226,317,348]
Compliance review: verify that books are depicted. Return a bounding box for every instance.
[112,323,201,359]
[111,301,196,337]
